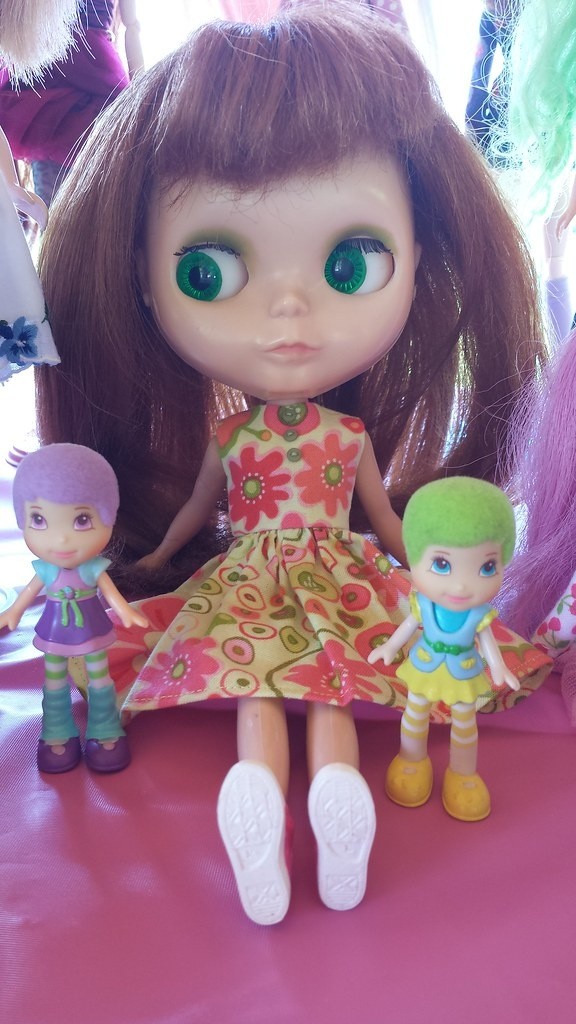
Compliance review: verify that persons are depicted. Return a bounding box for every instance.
[36,0,550,927]
[0,0,148,386]
[368,476,525,822]
[0,442,151,779]
[475,2,575,640]
